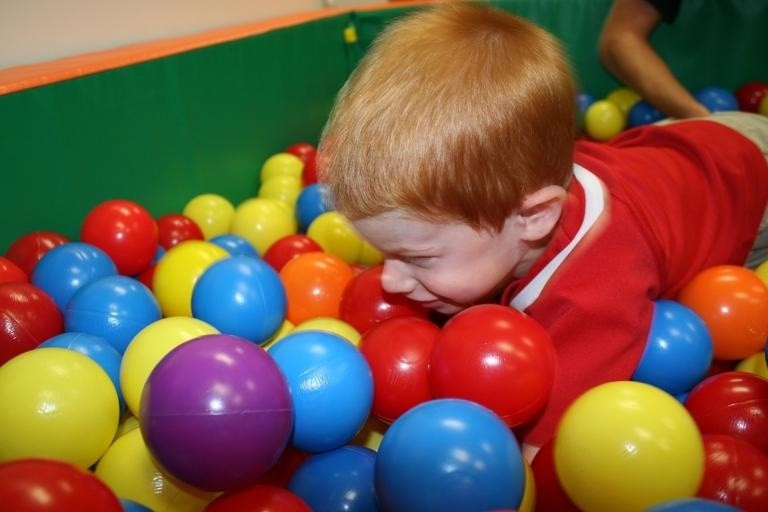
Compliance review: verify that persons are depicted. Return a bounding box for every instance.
[316,0,768,465]
[598,0,714,118]
